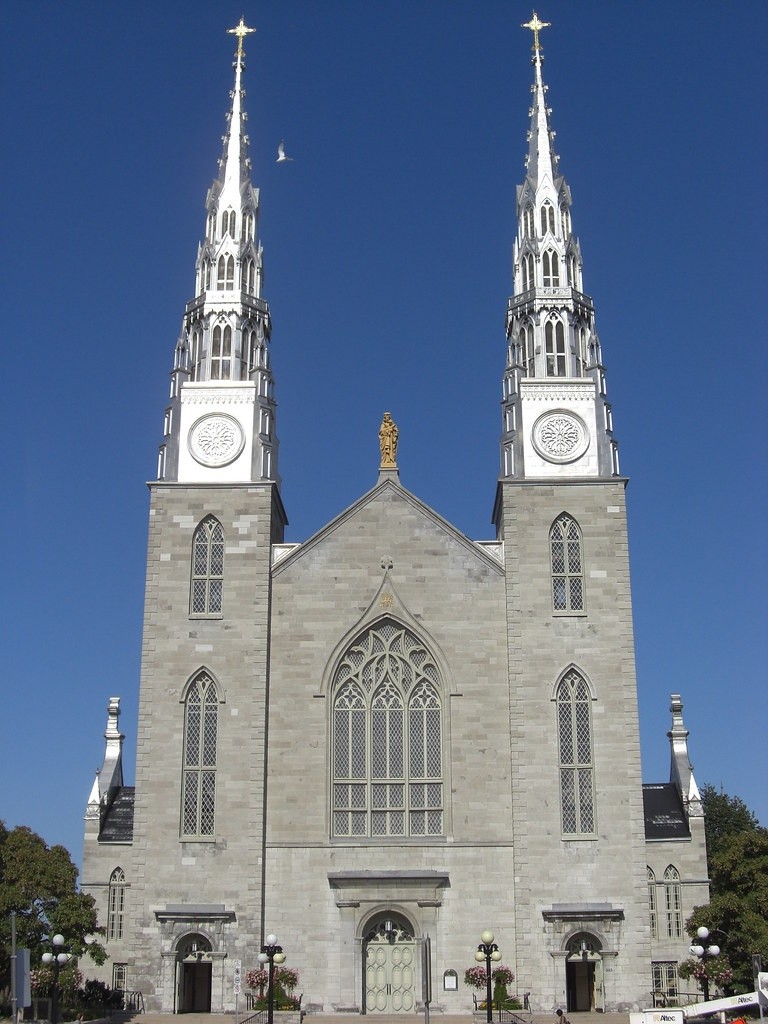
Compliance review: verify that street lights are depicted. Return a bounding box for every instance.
[689,928,721,1002]
[256,933,288,1024]
[41,934,73,1024]
[472,931,501,1024]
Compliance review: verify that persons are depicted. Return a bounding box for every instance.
[378,411,398,463]
[556,1009,567,1024]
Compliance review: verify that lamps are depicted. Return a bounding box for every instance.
[190,939,199,951]
[580,938,588,953]
[385,918,394,933]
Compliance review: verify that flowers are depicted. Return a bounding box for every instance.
[491,964,517,986]
[464,966,487,990]
[273,966,302,988]
[246,968,268,990]
